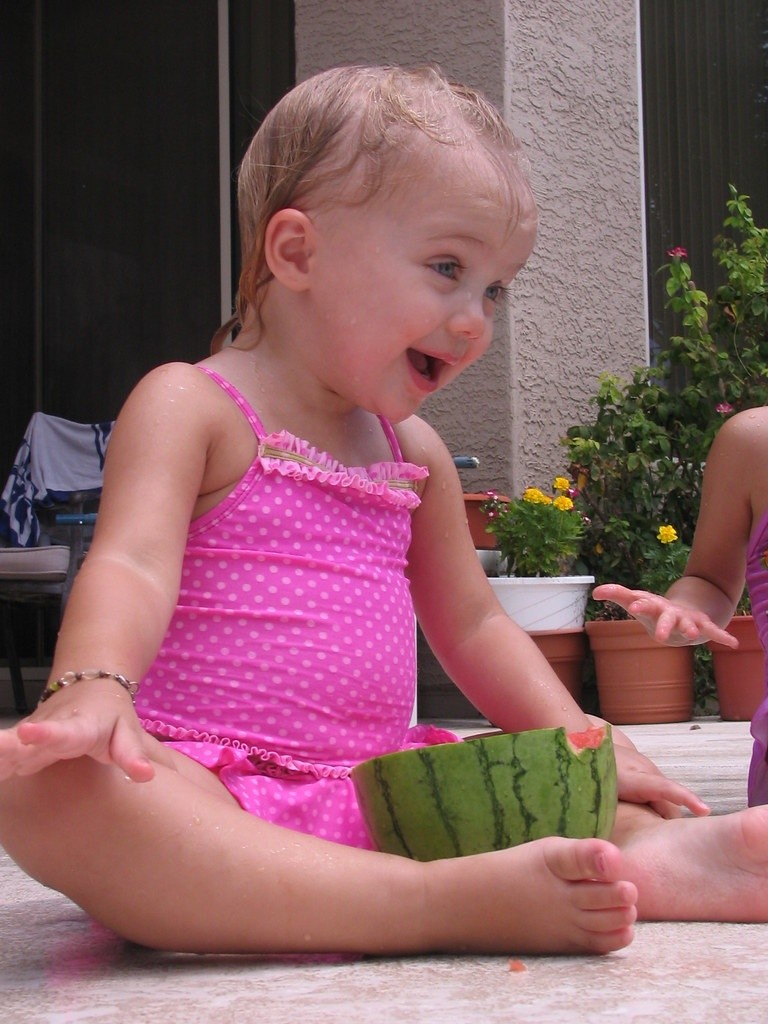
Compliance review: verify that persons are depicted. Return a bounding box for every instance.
[592,404,767,810]
[1,65,767,957]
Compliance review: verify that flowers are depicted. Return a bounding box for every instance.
[480,479,588,578]
[575,185,768,614]
[612,521,689,622]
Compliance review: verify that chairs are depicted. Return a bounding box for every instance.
[0,413,117,709]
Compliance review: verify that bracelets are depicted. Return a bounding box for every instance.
[37,669,140,706]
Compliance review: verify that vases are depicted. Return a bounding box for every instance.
[489,577,594,632]
[463,492,512,549]
[707,616,767,722]
[586,619,695,724]
[525,627,586,714]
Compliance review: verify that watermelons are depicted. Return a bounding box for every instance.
[351,724,618,862]
